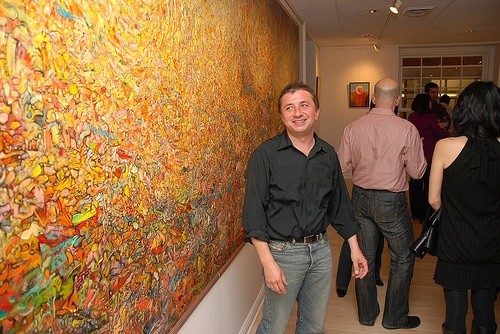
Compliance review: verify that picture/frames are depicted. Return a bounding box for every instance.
[349,82,370,107]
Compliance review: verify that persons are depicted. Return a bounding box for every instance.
[337,77,427,329]
[425,81,451,131]
[439,95,452,118]
[242,81,368,334]
[335,99,399,298]
[408,93,449,223]
[429,81,500,334]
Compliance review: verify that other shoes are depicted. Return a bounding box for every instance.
[383,316,421,330]
[335,287,347,297]
[360,301,380,326]
[375,277,384,286]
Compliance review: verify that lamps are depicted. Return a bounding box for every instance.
[373,44,381,52]
[389,0,402,15]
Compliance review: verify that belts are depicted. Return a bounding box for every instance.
[289,234,318,245]
